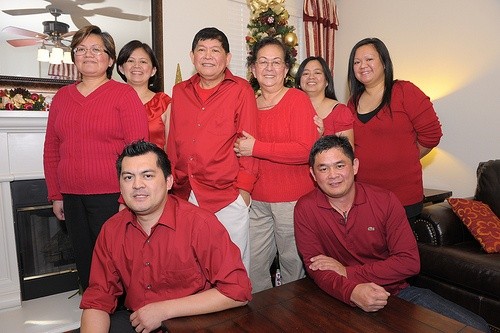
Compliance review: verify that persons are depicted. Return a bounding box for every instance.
[233,36,320,295]
[43,25,149,311]
[294,134,420,312]
[79,137,253,333]
[295,56,354,153]
[347,37,442,231]
[117,40,172,153]
[166,27,259,278]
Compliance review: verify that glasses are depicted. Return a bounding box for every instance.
[72,45,115,61]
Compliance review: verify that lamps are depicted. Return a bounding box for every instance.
[36,39,74,63]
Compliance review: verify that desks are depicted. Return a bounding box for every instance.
[423,187,452,204]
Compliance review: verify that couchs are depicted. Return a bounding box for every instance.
[405,159,500,328]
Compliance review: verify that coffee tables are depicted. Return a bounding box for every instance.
[163,275,489,333]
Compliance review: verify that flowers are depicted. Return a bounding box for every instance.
[0,87,50,111]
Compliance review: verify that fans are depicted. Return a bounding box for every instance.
[6,7,78,51]
